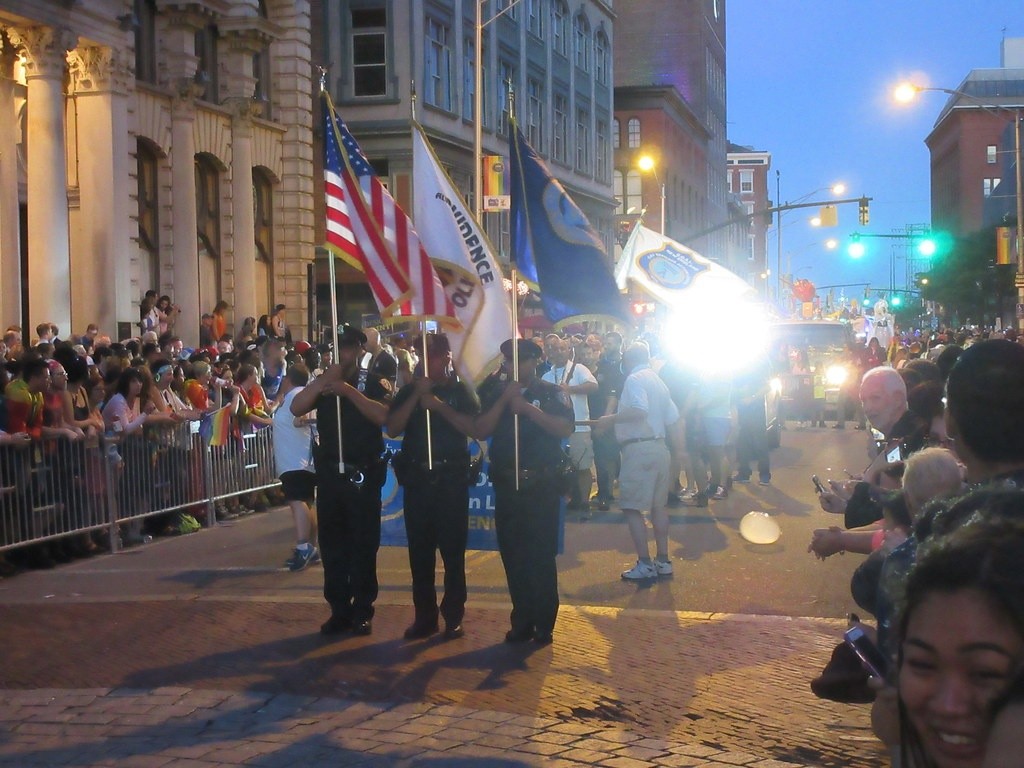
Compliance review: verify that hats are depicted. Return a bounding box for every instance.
[413,334,452,352]
[325,325,367,346]
[499,339,543,361]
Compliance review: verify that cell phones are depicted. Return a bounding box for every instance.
[812,475,826,494]
[845,625,889,677]
[886,445,902,462]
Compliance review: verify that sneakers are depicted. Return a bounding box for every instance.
[653,555,674,574]
[290,542,317,571]
[285,549,322,565]
[621,560,658,579]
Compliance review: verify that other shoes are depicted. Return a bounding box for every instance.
[321,613,355,633]
[445,615,465,637]
[506,625,535,640]
[126,486,284,541]
[675,469,771,507]
[534,623,554,643]
[797,419,866,429]
[0,531,123,578]
[580,501,589,513]
[405,622,439,639]
[598,501,614,509]
[353,617,372,635]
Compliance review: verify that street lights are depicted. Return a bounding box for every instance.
[889,81,1024,340]
[772,170,847,307]
[635,151,674,238]
[849,227,935,320]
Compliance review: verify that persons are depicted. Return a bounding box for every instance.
[0,289,1024,768]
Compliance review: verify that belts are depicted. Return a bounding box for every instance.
[619,435,666,448]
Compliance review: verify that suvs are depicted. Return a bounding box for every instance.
[753,322,865,431]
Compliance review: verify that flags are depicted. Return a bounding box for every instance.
[312,84,748,391]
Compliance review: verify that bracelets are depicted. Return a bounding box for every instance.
[839,550,845,555]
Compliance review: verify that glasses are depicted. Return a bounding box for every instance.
[55,372,68,376]
